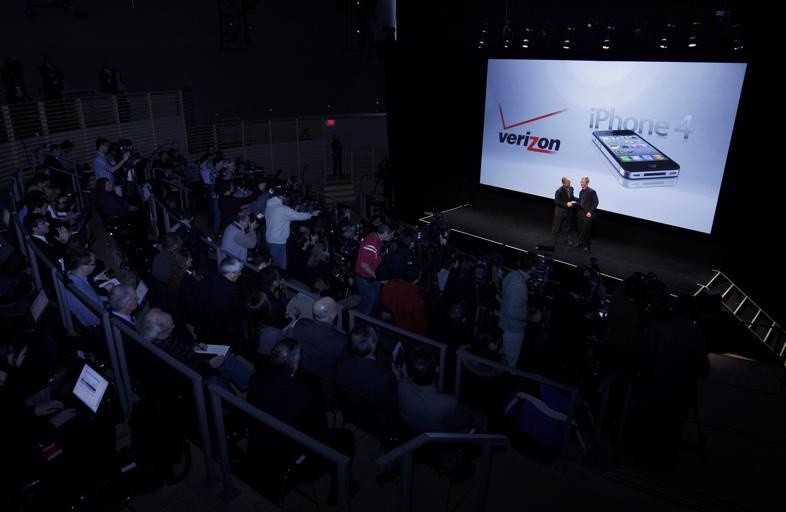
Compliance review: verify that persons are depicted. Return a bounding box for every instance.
[7,56,64,132]
[111,70,132,123]
[2,138,540,509]
[551,175,599,251]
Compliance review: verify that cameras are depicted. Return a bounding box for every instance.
[247,212,265,227]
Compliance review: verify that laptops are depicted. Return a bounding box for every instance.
[135,279,150,306]
[45,362,114,428]
[28,287,52,324]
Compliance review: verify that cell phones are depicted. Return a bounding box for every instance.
[591,130,680,180]
